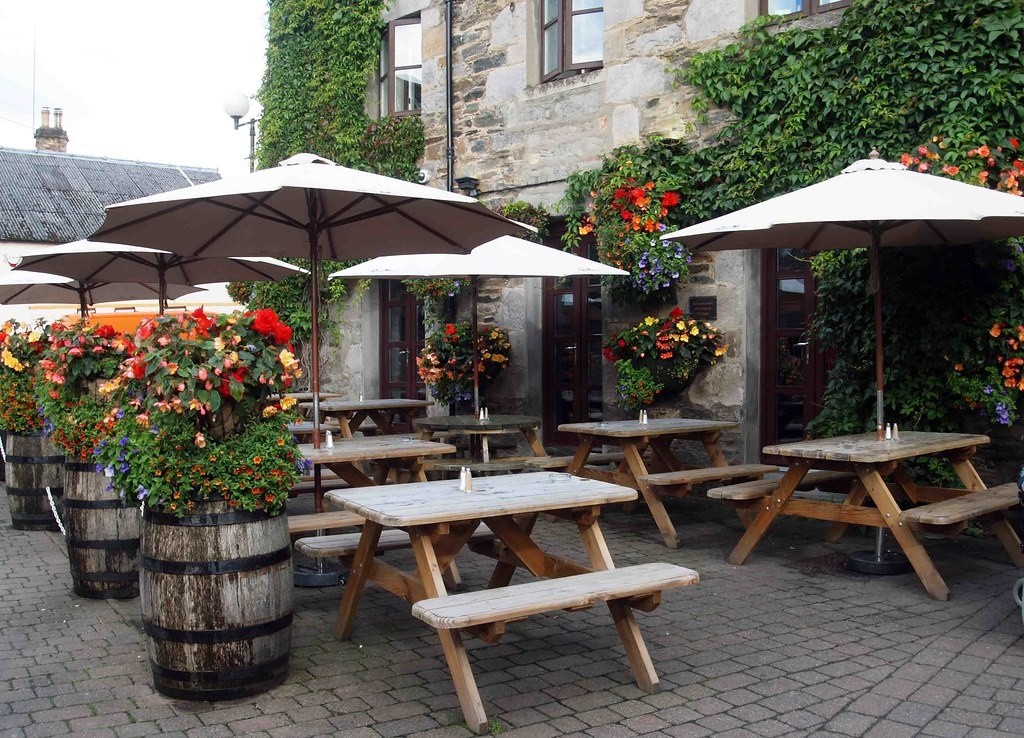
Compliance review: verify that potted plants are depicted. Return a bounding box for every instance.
[902,456,968,539]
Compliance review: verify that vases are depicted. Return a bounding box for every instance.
[62,448,142,599]
[5,424,65,531]
[139,479,294,702]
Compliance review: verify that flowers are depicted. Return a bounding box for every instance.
[901,133,1024,272]
[0,304,314,518]
[614,359,665,414]
[401,278,485,334]
[416,312,512,410]
[937,294,1024,427]
[579,161,693,306]
[602,306,730,391]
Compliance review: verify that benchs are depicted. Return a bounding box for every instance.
[301,469,342,482]
[412,562,699,630]
[524,452,626,468]
[405,432,449,438]
[422,457,552,471]
[287,510,367,532]
[638,464,779,485]
[454,445,517,451]
[324,420,351,426]
[707,470,858,500]
[294,522,497,558]
[355,422,402,430]
[899,483,1024,524]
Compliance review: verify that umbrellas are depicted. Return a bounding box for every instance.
[329,235,630,461]
[0,238,312,316]
[88,153,539,511]
[659,147,1024,556]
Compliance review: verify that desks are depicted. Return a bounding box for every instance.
[324,471,661,736]
[728,430,1024,601]
[298,399,435,435]
[266,393,342,422]
[558,418,760,553]
[297,434,457,532]
[412,415,556,476]
[287,424,336,444]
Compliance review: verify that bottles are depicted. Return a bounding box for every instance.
[357,392,363,402]
[885,423,892,440]
[893,423,898,439]
[459,467,466,491]
[480,408,484,419]
[464,468,472,493]
[325,430,333,448]
[643,410,647,424]
[485,407,488,418]
[639,410,643,424]
